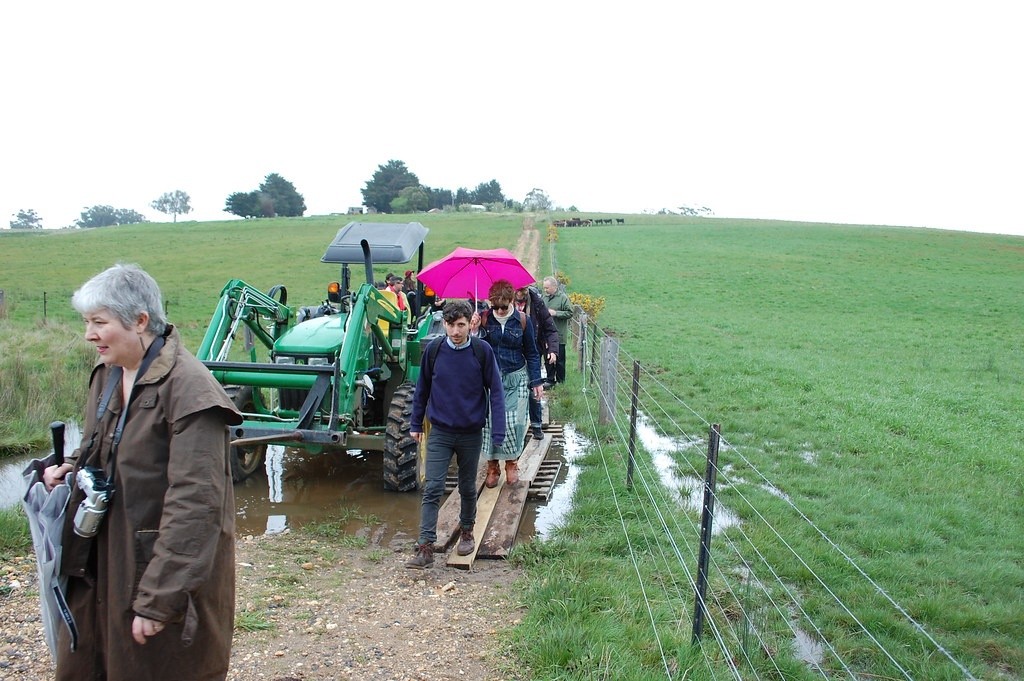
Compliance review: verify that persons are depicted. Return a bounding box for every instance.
[470,280,544,488]
[385,273,395,288]
[44,265,245,681]
[385,277,411,327]
[511,286,558,440]
[403,299,507,570]
[542,277,573,390]
[402,270,416,293]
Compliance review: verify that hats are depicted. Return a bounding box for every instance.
[405,270,414,279]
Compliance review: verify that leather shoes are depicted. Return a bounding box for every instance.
[531,422,544,440]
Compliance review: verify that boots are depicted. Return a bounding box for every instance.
[405,538,434,569]
[505,459,519,486]
[486,460,501,488]
[457,521,474,555]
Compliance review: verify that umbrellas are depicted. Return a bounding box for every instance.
[415,246,537,313]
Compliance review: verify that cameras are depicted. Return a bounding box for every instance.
[73,465,116,539]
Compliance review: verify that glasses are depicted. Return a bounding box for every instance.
[396,282,404,285]
[491,301,511,310]
[514,296,526,303]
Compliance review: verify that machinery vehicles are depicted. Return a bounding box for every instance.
[186,217,448,496]
